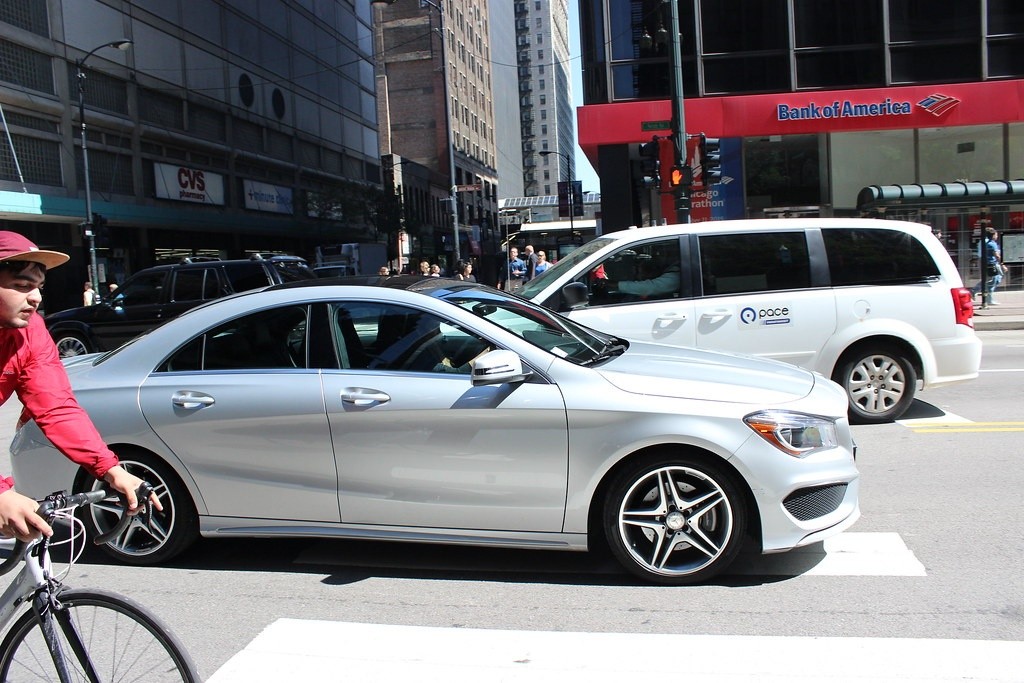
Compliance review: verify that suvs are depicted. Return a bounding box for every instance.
[353,216,985,427]
[42,254,318,360]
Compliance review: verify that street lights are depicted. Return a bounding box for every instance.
[477,194,494,265]
[73,37,135,295]
[537,151,573,243]
[381,159,411,269]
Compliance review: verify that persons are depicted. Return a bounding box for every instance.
[420,261,430,276]
[598,252,681,304]
[971,227,1004,305]
[498,247,527,293]
[109,284,124,298]
[456,262,476,282]
[0,229,164,541]
[380,267,389,275]
[430,264,441,277]
[524,244,538,280]
[535,251,552,276]
[84,281,96,306]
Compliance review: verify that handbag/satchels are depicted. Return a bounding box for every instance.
[987,263,998,276]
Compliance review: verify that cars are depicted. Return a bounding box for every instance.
[9,275,862,587]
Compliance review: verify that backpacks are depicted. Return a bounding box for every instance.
[86,290,102,305]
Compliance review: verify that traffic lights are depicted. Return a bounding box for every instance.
[697,132,722,186]
[94,213,108,251]
[82,223,97,236]
[638,140,662,191]
[671,166,692,186]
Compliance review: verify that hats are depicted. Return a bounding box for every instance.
[0,231,70,270]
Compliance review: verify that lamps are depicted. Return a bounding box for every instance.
[640,9,668,50]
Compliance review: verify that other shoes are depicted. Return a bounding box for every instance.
[969,290,976,301]
[987,302,1000,305]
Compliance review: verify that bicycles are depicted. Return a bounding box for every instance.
[0,478,206,683]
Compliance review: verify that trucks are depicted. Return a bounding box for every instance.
[313,243,387,279]
